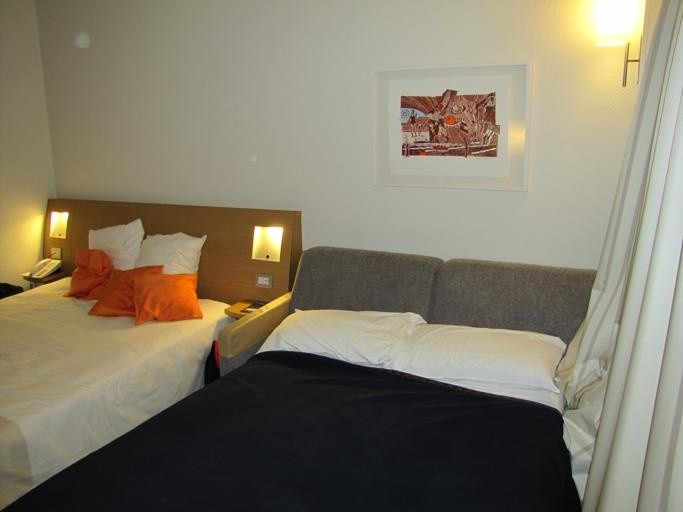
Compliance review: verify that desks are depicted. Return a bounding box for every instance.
[225,300,268,318]
[0,246,601,512]
[23,271,66,283]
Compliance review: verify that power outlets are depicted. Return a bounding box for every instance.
[254,273,273,289]
[49,247,62,261]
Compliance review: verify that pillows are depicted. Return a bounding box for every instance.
[63,216,208,326]
[259,308,427,395]
[383,322,568,395]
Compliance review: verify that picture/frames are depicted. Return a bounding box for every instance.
[370,59,533,193]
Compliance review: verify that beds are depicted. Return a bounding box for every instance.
[0,199,303,512]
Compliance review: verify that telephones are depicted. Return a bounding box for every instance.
[29,258,62,279]
